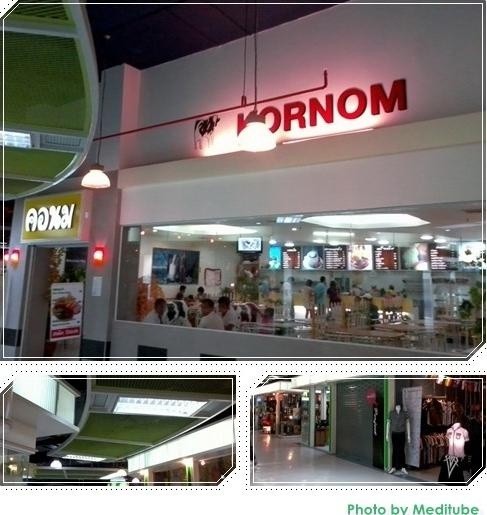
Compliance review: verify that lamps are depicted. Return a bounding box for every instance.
[92,247,105,262]
[131,471,141,483]
[81,5,112,190]
[235,4,277,152]
[11,250,19,262]
[50,452,61,469]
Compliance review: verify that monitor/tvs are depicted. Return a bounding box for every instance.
[236,237,263,254]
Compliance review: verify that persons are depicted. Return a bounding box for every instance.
[385,405,411,475]
[142,276,398,332]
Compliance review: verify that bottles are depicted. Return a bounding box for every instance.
[439,291,459,317]
[274,295,404,324]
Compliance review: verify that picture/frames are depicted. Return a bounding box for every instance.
[204,268,222,287]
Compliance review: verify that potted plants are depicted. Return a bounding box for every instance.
[44,246,67,357]
